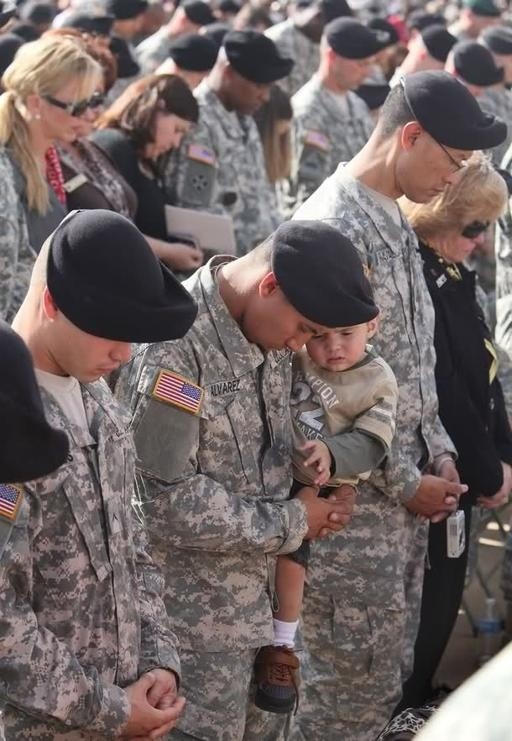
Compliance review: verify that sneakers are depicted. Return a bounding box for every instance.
[252,645,301,716]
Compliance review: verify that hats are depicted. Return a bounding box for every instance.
[12,25,40,41]
[453,43,505,89]
[400,70,507,153]
[222,28,298,85]
[419,19,460,59]
[49,7,118,36]
[326,18,374,62]
[0,37,15,65]
[0,320,71,486]
[274,219,380,328]
[45,211,199,345]
[109,33,140,78]
[184,1,215,24]
[364,19,397,48]
[482,23,512,55]
[170,32,219,74]
[467,0,499,15]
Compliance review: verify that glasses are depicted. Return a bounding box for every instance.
[41,91,112,116]
[432,135,468,178]
[457,219,493,243]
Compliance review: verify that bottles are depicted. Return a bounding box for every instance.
[478,599,502,665]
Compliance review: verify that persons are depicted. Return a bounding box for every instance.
[476,25,510,104]
[253,263,402,714]
[95,0,149,37]
[18,1,57,32]
[86,72,202,282]
[106,38,144,78]
[397,154,512,714]
[279,65,507,741]
[448,0,499,42]
[0,207,199,737]
[384,25,456,82]
[55,24,138,225]
[113,219,378,741]
[250,83,309,216]
[262,0,352,82]
[5,23,37,40]
[444,41,511,424]
[294,21,384,192]
[155,30,284,257]
[62,7,116,38]
[130,0,215,70]
[156,1,512,24]
[1,34,104,319]
[200,0,238,40]
[229,4,270,33]
